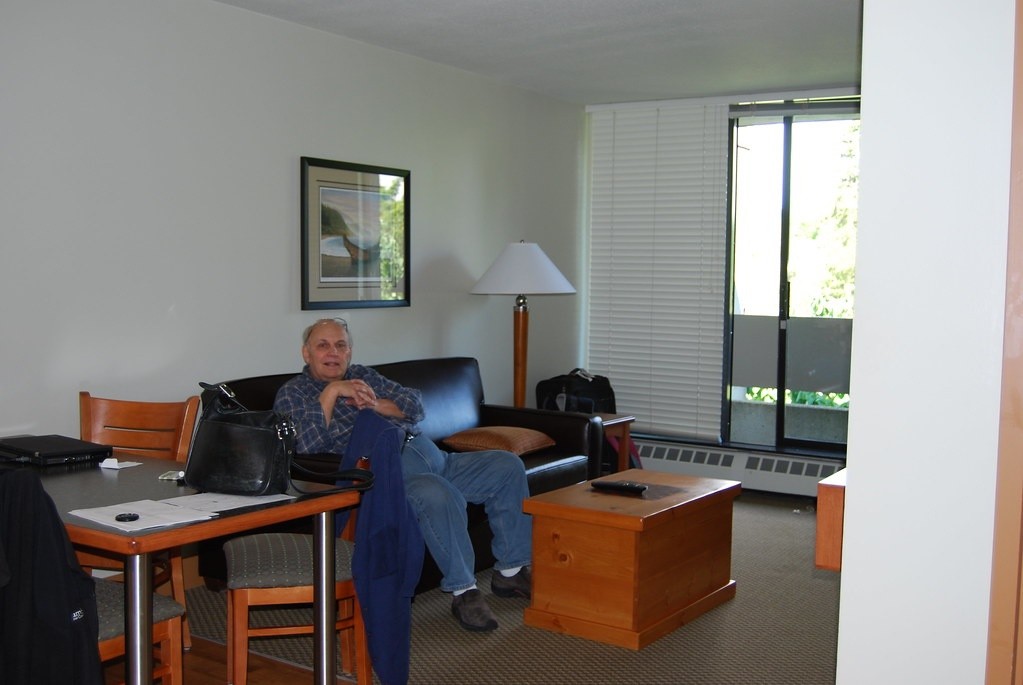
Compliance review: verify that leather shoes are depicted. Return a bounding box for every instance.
[491,566,531,599]
[452,589,498,631]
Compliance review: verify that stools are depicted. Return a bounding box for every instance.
[84,575,186,685]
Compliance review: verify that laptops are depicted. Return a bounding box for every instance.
[0,434,113,466]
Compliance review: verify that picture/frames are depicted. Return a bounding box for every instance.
[299,155,412,311]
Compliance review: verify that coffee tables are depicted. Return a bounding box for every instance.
[521,467,744,650]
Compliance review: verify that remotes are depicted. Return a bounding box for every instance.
[590,480,647,493]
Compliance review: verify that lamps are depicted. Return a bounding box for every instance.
[467,239,577,410]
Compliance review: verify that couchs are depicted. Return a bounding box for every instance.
[200,357,606,597]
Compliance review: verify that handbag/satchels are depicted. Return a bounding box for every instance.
[185,381,296,495]
[536,368,617,414]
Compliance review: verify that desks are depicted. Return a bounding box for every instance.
[32,452,361,685]
[585,413,639,473]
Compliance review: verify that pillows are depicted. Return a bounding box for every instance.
[439,424,556,460]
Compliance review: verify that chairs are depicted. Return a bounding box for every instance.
[219,452,374,685]
[67,389,201,653]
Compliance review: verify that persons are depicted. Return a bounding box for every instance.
[275,318,539,633]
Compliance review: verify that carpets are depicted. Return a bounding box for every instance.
[185,490,841,685]
